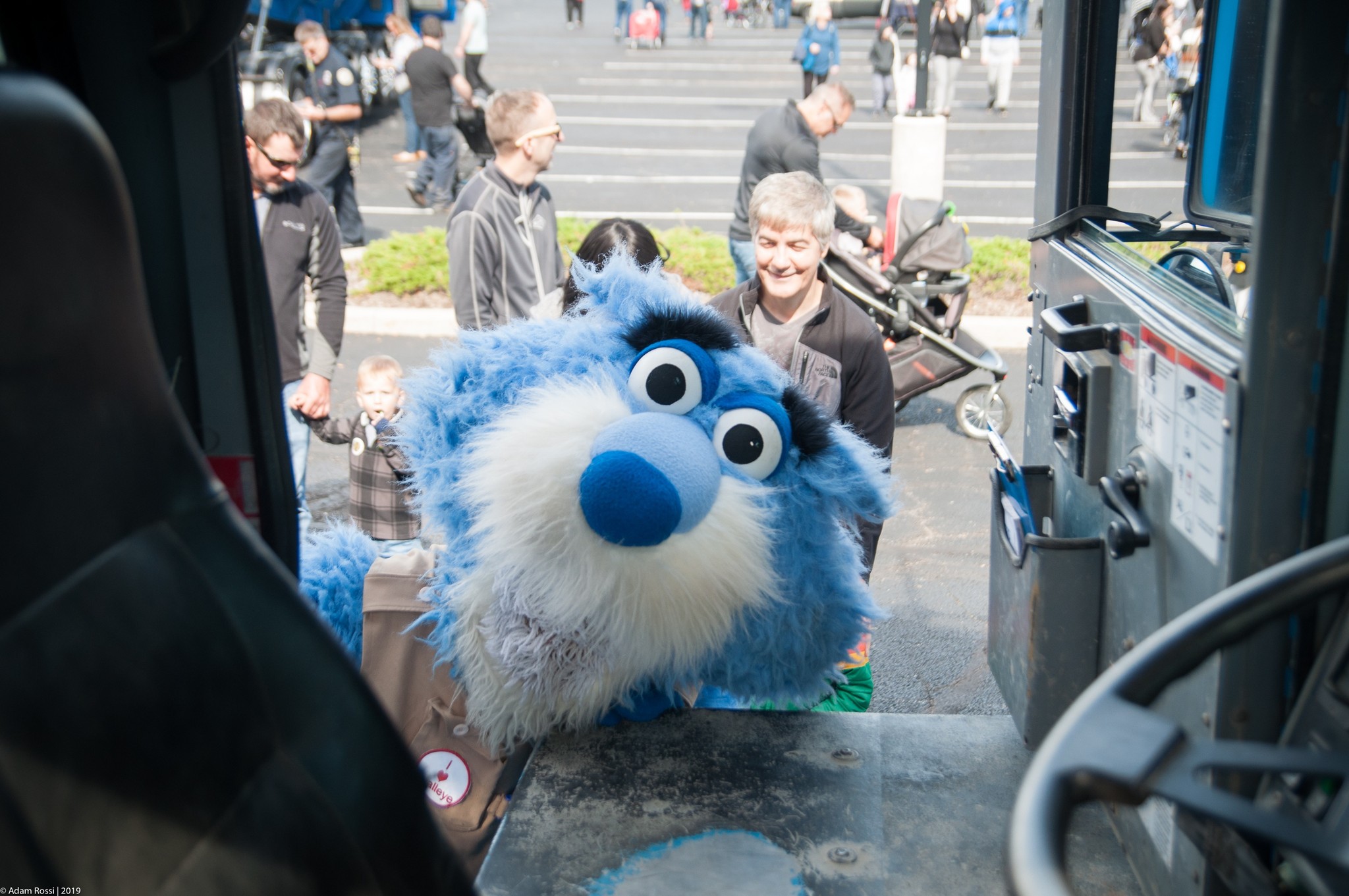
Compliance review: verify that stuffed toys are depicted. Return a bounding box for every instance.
[294,242,901,759]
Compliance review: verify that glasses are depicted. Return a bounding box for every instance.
[822,101,843,130]
[252,137,306,172]
[515,123,562,147]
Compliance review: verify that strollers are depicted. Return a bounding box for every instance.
[818,188,1014,440]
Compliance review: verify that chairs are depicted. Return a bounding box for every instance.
[0,78,478,896]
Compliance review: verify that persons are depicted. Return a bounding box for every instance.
[725,80,886,284]
[565,0,583,26]
[830,183,883,271]
[243,96,348,518]
[447,88,567,331]
[293,20,365,249]
[613,0,1030,118]
[1129,0,1204,159]
[368,0,499,207]
[532,218,664,321]
[750,576,874,713]
[704,170,896,586]
[297,352,421,541]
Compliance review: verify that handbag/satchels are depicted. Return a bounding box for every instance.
[1174,77,1190,94]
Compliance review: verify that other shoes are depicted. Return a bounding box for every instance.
[390,149,429,163]
[432,200,456,213]
[337,236,364,249]
[405,180,428,207]
